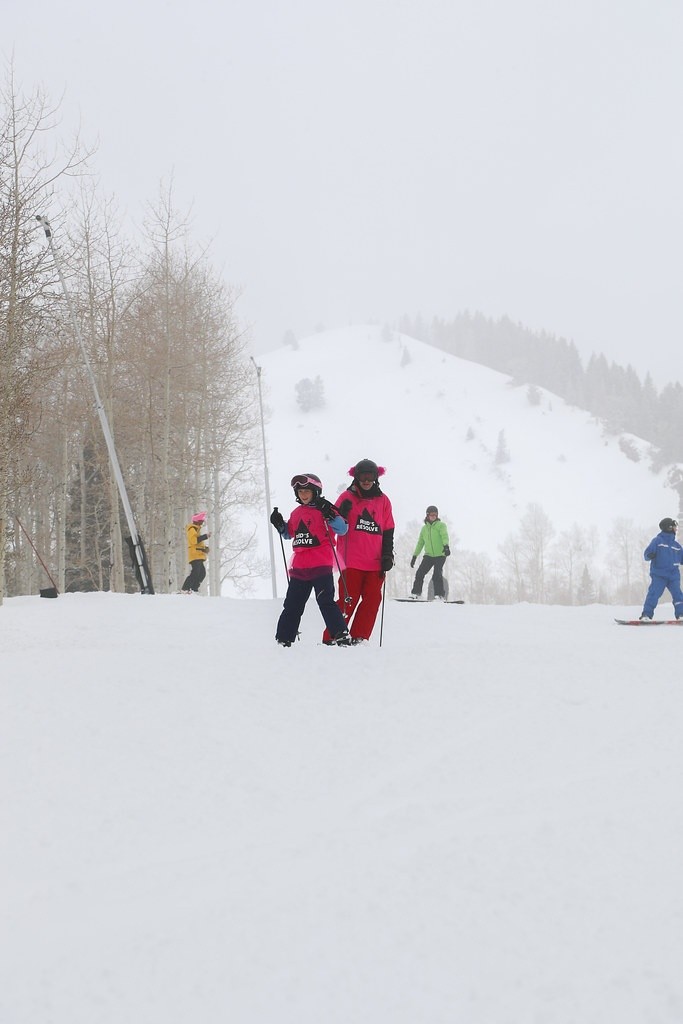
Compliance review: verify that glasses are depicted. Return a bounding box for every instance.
[291,475,310,487]
[355,471,376,482]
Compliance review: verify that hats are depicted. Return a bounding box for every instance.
[349,459,385,478]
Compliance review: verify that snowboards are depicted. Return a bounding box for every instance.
[394,597,465,604]
[614,617,683,626]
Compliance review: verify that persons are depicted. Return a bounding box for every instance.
[322,458,395,646]
[269,474,364,647]
[180,512,212,595]
[408,506,450,602]
[639,518,683,620]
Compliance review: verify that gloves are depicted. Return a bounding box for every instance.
[339,499,352,516]
[314,497,333,517]
[442,546,450,556]
[206,531,211,538]
[646,553,655,559]
[411,556,416,567]
[382,555,394,571]
[271,507,284,530]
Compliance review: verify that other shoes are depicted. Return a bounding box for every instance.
[434,595,444,601]
[409,594,418,599]
[277,638,291,647]
[335,632,356,646]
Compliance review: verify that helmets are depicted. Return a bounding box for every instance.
[426,506,438,515]
[295,473,322,495]
[659,517,675,534]
